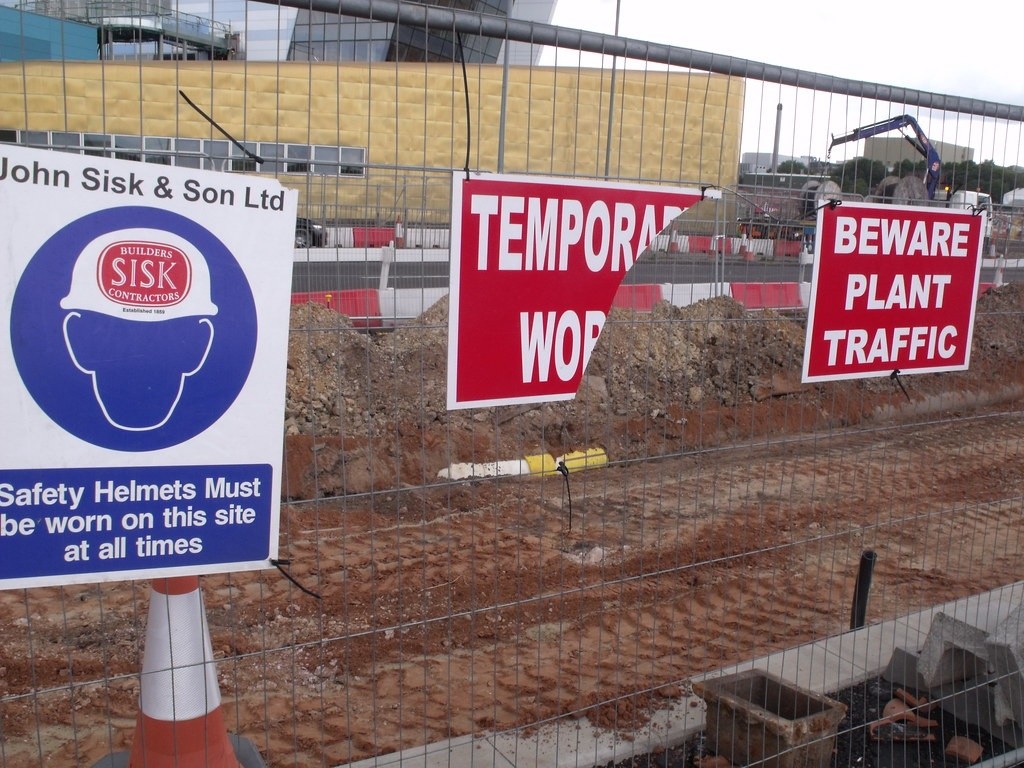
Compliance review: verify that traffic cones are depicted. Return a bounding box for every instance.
[988,232,997,259]
[993,254,1006,287]
[86,570,267,767]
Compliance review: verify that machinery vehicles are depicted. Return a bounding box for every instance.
[827,114,942,201]
